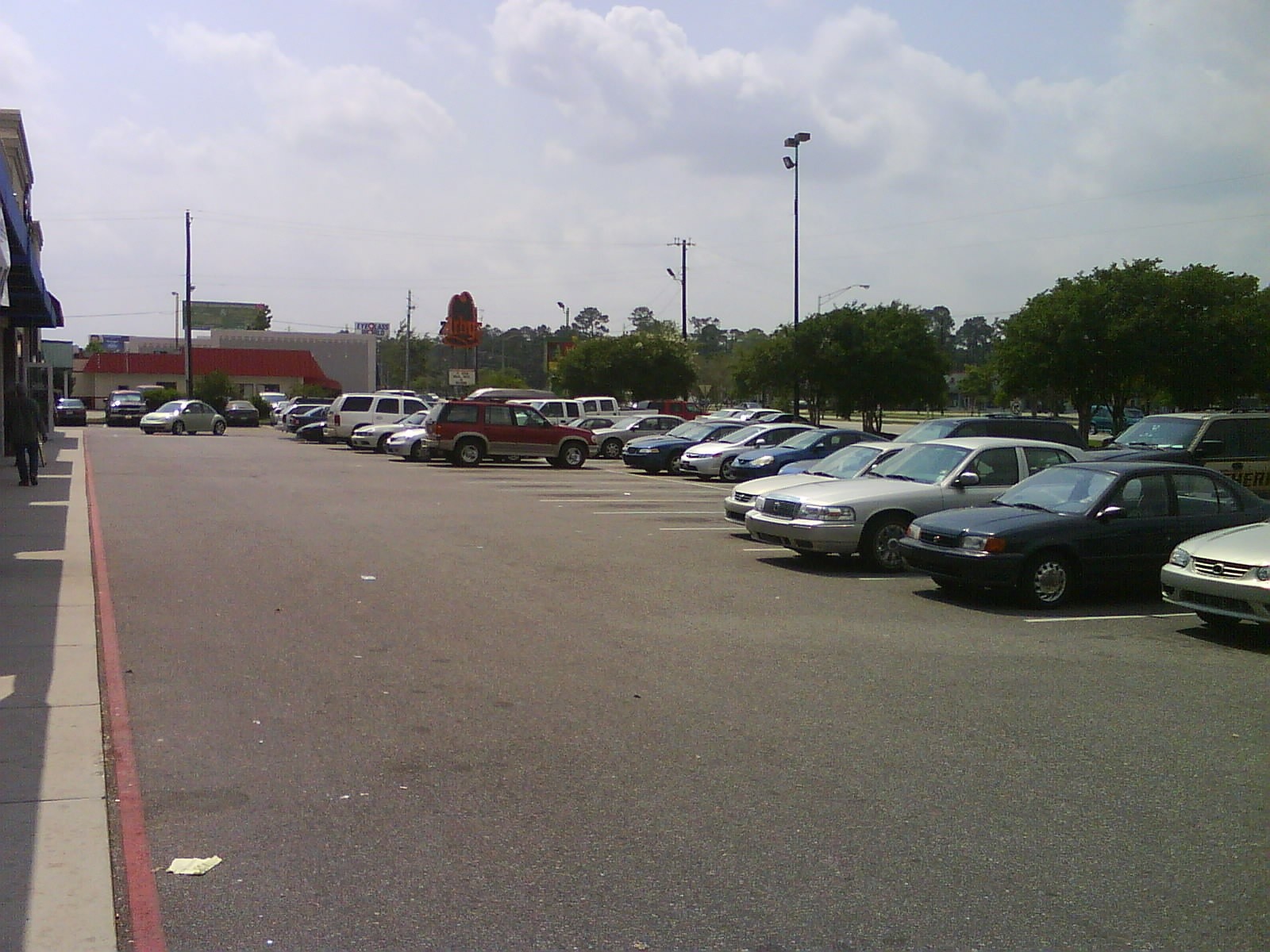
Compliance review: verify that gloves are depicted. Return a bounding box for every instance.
[42,436,48,443]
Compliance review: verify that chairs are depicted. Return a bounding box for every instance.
[1124,479,1151,509]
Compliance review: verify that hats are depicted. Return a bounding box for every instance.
[17,383,27,394]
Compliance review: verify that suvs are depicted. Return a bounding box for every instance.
[646,400,712,421]
[420,397,592,471]
[779,411,1083,476]
[1084,406,1270,502]
[463,387,614,459]
[104,390,149,427]
[1089,404,1161,436]
[323,392,432,450]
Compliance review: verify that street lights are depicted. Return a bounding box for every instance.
[555,301,569,327]
[781,131,812,422]
[171,291,179,348]
[818,283,870,315]
[665,267,688,400]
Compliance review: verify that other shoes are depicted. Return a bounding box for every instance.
[19,477,29,486]
[14,461,18,466]
[30,477,38,485]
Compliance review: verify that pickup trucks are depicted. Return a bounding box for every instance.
[575,396,660,416]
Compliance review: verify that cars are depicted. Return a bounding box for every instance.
[1159,519,1270,637]
[680,422,841,483]
[258,392,337,445]
[745,436,1102,573]
[591,413,686,461]
[139,399,226,435]
[896,460,1270,611]
[695,402,839,430]
[350,411,432,455]
[379,389,440,408]
[56,398,88,426]
[226,400,259,427]
[724,441,992,524]
[384,428,433,463]
[729,427,895,481]
[621,420,745,476]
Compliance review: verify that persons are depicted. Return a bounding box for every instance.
[4,384,47,485]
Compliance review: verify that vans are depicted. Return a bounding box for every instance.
[134,385,165,394]
